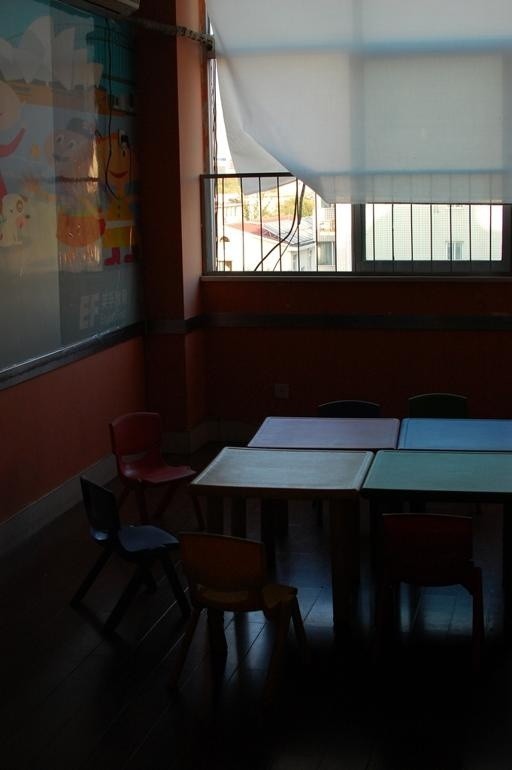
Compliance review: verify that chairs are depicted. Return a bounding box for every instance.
[394,391,487,519]
[105,408,196,524]
[173,531,311,677]
[69,473,195,637]
[311,400,386,525]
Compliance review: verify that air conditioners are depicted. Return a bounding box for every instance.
[66,0,142,20]
